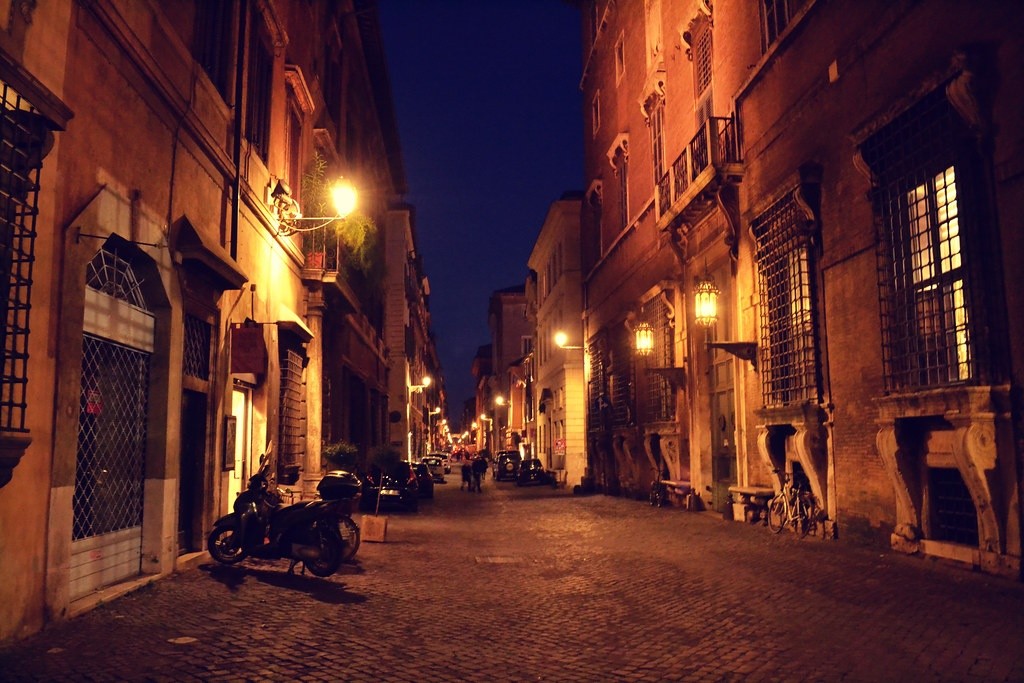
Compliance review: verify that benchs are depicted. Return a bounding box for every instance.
[661,479,690,496]
[728,486,774,507]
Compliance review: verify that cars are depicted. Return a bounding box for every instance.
[420,456,445,482]
[358,459,421,514]
[429,453,452,474]
[515,459,546,486]
[412,461,434,498]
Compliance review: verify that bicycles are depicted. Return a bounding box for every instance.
[647,467,667,508]
[767,469,815,538]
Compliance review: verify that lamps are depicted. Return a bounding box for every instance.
[634,306,656,357]
[691,256,721,330]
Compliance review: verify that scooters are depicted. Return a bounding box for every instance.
[206,439,364,578]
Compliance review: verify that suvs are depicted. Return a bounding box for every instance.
[492,450,522,481]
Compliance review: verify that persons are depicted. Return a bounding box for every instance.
[458,454,488,496]
